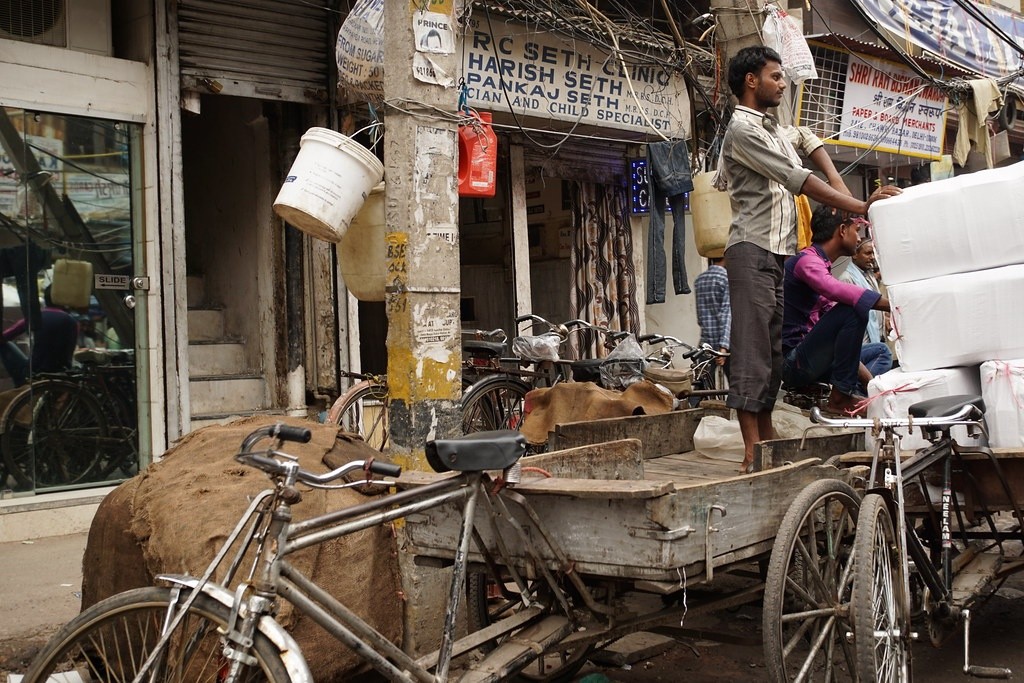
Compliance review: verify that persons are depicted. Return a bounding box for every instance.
[781,202,894,417]
[722,47,904,473]
[694,257,733,389]
[838,237,884,343]
[2,283,80,389]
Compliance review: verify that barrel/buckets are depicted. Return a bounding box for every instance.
[271,123,386,245]
[335,180,386,302]
[459,108,497,198]
[51,260,93,308]
[690,169,733,259]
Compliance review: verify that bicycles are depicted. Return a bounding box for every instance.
[0,344,140,496]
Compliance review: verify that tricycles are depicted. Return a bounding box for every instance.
[313,308,1023,683]
[19,392,873,683]
